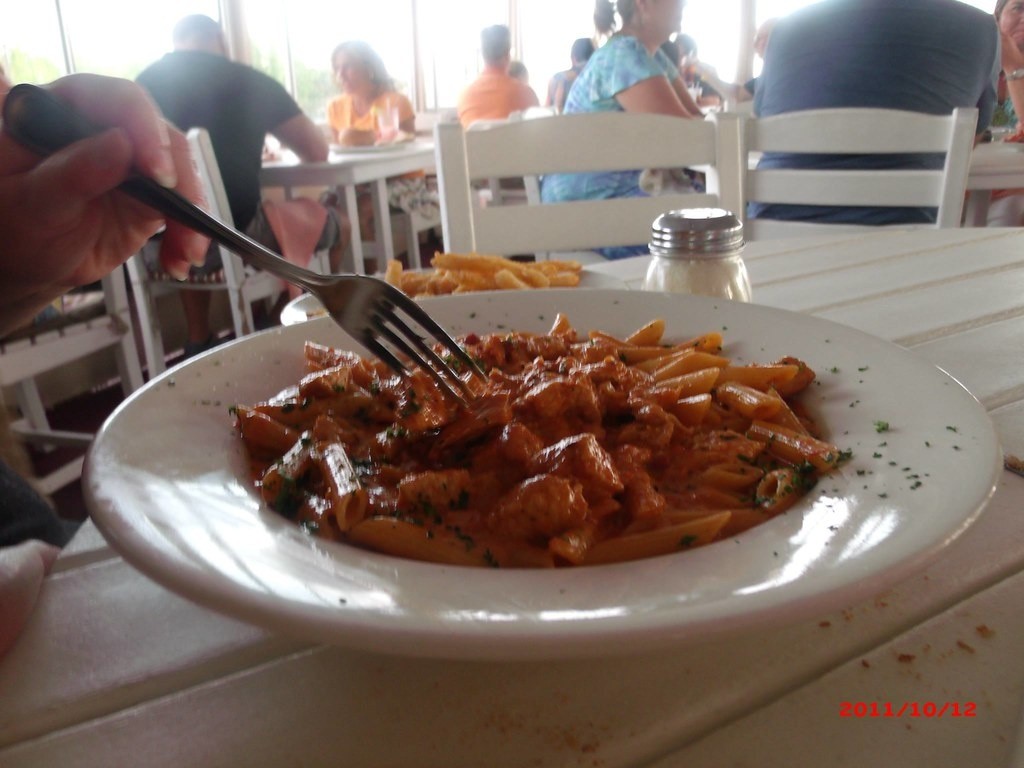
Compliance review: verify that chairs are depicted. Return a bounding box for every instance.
[2,86,1023,517]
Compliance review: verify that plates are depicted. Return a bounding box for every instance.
[279,269,629,327]
[82,288,1005,658]
[328,145,404,153]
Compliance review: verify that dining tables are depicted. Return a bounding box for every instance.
[2,223,1024,767]
[251,133,434,272]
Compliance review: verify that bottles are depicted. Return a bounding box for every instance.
[643,208,753,303]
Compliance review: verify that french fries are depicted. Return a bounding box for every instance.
[384,250,584,297]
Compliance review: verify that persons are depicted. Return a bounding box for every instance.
[123,0,1024,360]
[0,74,212,675]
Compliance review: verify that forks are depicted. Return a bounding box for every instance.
[3,84,489,410]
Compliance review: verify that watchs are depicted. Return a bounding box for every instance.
[1007,69,1024,83]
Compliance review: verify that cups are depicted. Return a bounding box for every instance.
[379,107,399,143]
[681,59,697,87]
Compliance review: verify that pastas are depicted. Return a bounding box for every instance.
[227,312,843,567]
